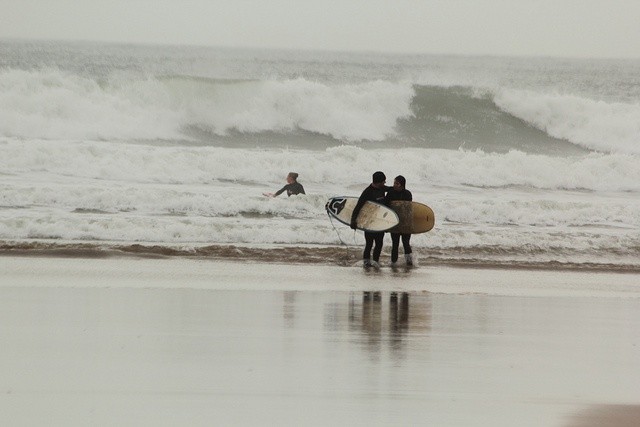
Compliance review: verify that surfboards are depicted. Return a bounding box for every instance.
[263,192,276,198]
[326,195,399,232]
[387,201,434,234]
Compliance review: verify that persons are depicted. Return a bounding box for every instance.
[272,172,306,198]
[386,175,413,266]
[350,171,393,266]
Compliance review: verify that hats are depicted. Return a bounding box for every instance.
[373,172,386,183]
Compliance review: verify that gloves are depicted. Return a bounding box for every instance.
[351,222,356,229]
